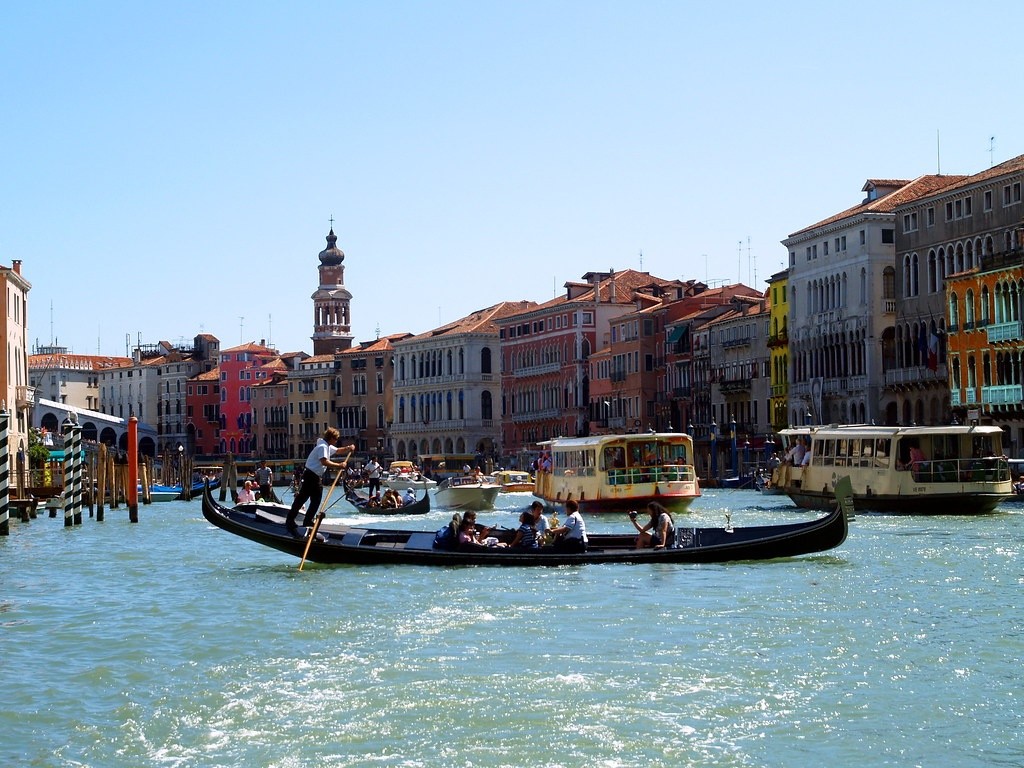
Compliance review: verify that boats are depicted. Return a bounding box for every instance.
[291,483,301,492]
[382,470,437,490]
[343,478,430,515]
[138,491,181,502]
[201,478,850,566]
[532,431,702,514]
[10,506,47,518]
[490,471,535,492]
[756,425,1024,514]
[434,476,502,510]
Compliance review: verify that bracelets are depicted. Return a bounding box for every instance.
[347,445,350,452]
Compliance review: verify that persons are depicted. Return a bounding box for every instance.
[607,457,688,484]
[510,500,588,552]
[783,437,810,466]
[286,426,356,539]
[346,455,383,499]
[237,459,273,503]
[346,467,355,479]
[391,465,422,481]
[984,452,1009,474]
[368,487,417,509]
[463,462,471,475]
[531,451,552,477]
[965,461,976,480]
[971,444,982,458]
[192,470,216,484]
[290,473,299,492]
[896,440,927,481]
[470,465,488,483]
[627,501,675,550]
[442,509,511,548]
[1014,476,1024,492]
[756,451,780,488]
[423,466,440,485]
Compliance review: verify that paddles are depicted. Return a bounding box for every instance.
[282,485,293,495]
[729,470,765,495]
[299,452,351,570]
[323,466,380,513]
[242,474,249,490]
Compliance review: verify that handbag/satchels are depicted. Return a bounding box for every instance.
[665,532,674,546]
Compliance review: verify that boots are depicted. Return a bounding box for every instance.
[286,509,298,528]
[303,510,314,526]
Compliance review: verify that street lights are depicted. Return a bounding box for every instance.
[178,445,184,487]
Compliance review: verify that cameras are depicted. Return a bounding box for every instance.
[630,510,637,519]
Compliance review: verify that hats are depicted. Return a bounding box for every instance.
[408,488,413,491]
